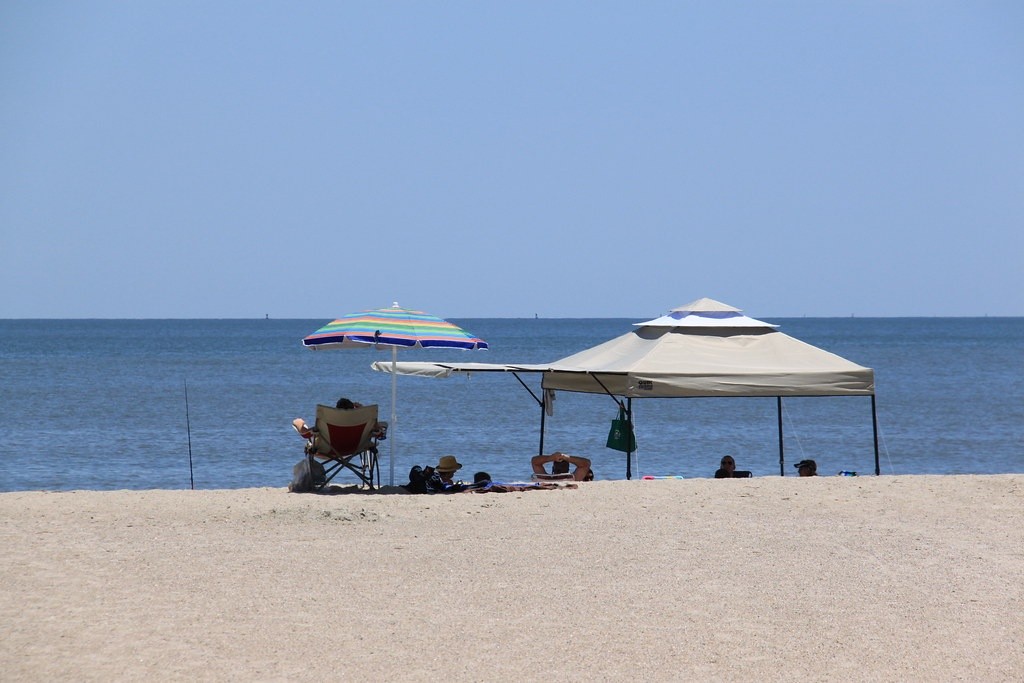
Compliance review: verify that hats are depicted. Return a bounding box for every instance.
[436,455,462,473]
[794,461,810,468]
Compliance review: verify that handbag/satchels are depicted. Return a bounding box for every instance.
[606,399,637,452]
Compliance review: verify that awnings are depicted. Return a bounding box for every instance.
[373,359,626,417]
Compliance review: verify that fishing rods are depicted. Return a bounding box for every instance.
[183,378,195,490]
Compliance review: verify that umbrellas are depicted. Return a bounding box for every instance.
[300,301,488,485]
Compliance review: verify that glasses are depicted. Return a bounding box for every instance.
[721,461,733,465]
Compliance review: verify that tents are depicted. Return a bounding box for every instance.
[535,294,881,480]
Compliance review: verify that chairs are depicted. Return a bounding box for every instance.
[531,473,575,482]
[642,476,683,480]
[291,404,388,489]
[715,469,753,479]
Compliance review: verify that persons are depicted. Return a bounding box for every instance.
[293,397,387,460]
[474,473,493,488]
[794,459,819,476]
[713,455,741,478]
[531,451,591,480]
[405,456,462,490]
